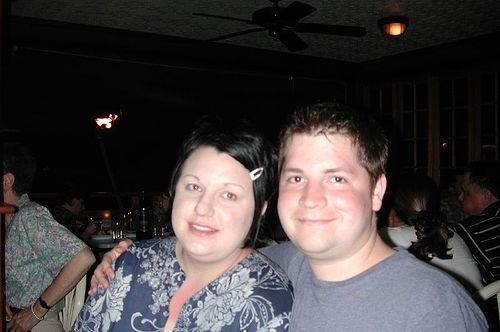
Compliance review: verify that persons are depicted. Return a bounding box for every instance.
[380,160,499,287]
[72,115,294,332]
[1,148,96,332]
[46,190,101,241]
[93,96,489,332]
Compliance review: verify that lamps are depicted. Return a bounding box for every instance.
[377,15,409,36]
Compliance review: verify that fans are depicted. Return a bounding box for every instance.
[192,0,367,52]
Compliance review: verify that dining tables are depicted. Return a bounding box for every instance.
[85,225,137,258]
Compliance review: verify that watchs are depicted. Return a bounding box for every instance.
[38,297,51,310]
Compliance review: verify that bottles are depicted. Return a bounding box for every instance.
[140,190,148,234]
[153,201,165,238]
[123,207,134,233]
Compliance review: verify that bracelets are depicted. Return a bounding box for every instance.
[30,303,44,322]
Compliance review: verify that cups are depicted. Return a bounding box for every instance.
[90,217,103,236]
[112,226,123,243]
